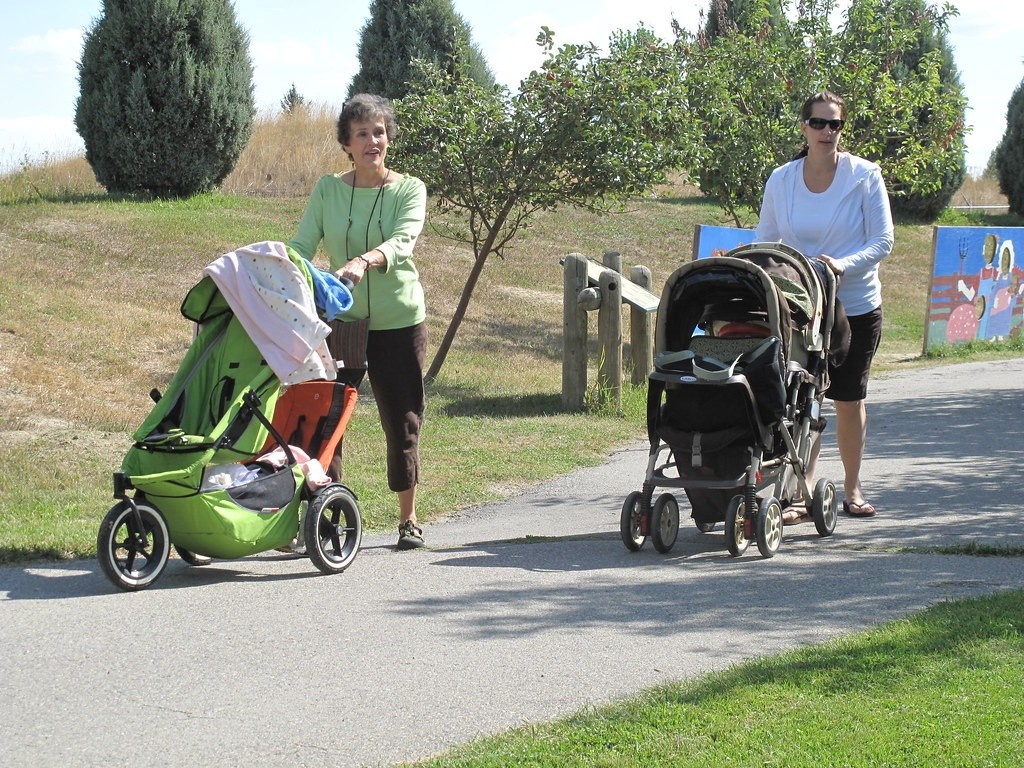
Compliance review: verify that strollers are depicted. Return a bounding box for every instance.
[617,241,841,556]
[97,240,369,593]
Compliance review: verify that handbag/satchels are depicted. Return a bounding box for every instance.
[328,317,369,370]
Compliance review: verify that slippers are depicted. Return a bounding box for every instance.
[782,497,813,524]
[842,500,877,517]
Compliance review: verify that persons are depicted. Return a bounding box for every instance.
[275,92,428,555]
[756,91,896,525]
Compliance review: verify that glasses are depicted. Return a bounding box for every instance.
[805,118,846,131]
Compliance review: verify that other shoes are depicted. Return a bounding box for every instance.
[275,538,308,555]
[397,520,423,549]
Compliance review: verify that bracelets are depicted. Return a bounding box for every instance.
[358,255,369,270]
